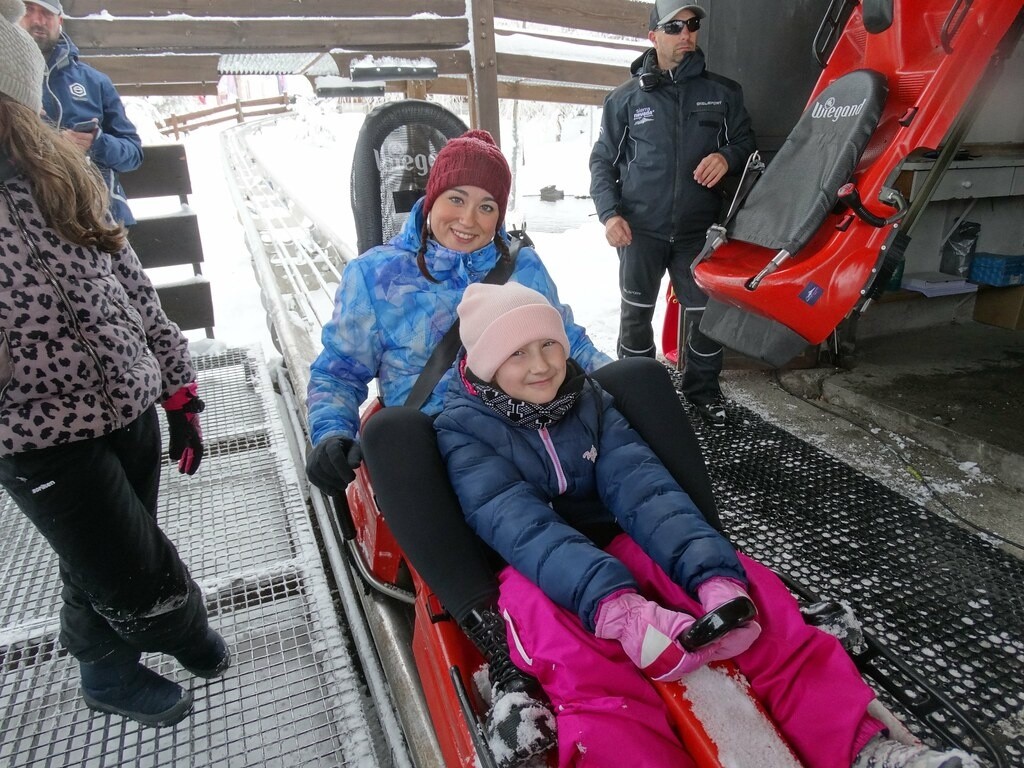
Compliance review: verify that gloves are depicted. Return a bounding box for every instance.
[304,434,363,501]
[593,590,721,685]
[695,574,764,662]
[161,377,209,478]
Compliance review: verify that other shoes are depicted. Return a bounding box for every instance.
[696,399,730,430]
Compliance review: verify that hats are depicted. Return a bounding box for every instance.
[0,0,46,116]
[21,0,63,15]
[423,129,513,232]
[454,280,571,381]
[649,0,707,31]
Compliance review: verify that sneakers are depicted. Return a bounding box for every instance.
[855,731,966,768]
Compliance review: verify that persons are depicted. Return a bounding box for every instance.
[588,0,756,428]
[20,0,143,225]
[0,0,229,727]
[435,279,972,768]
[305,130,733,768]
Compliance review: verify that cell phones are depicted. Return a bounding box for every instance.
[74,120,97,133]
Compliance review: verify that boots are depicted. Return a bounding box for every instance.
[151,607,233,680]
[457,595,560,768]
[794,597,865,662]
[80,649,195,730]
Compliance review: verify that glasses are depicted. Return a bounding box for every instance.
[655,16,702,36]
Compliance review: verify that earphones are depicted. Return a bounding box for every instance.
[59,25,63,33]
